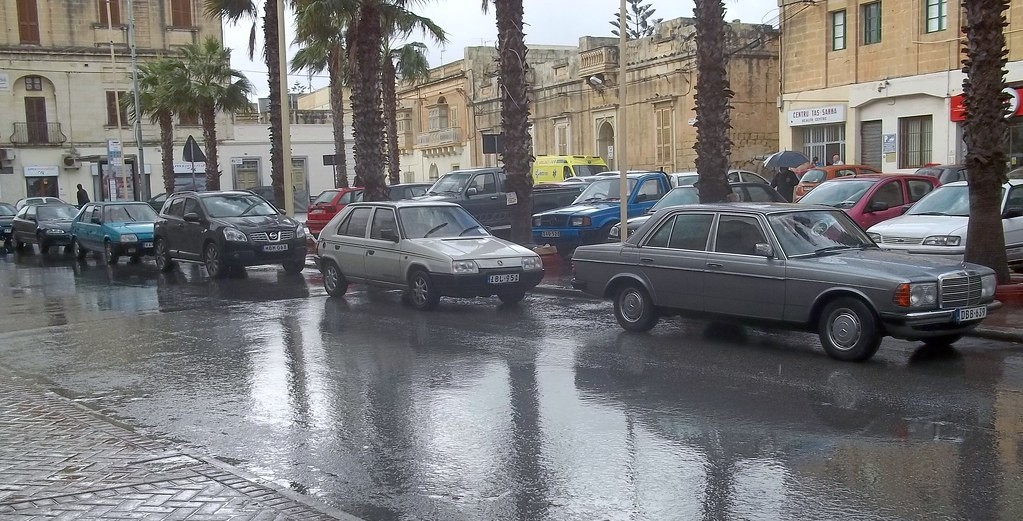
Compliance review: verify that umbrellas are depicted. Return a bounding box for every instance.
[763,148,810,168]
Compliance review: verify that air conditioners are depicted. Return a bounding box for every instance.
[61,153,82,169]
[0,148,15,161]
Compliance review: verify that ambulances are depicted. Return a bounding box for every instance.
[533,155,611,186]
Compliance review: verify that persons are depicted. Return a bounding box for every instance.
[77,184,90,209]
[832,155,844,165]
[812,157,823,167]
[771,167,799,203]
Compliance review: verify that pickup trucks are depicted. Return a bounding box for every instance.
[411,166,589,231]
[531,171,673,256]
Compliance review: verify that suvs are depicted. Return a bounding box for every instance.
[153,189,308,279]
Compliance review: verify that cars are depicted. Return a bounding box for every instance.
[596,171,649,175]
[70,201,160,264]
[914,164,968,184]
[792,163,884,201]
[360,182,433,202]
[306,187,363,236]
[0,202,20,239]
[865,177,1023,263]
[666,172,699,188]
[607,182,790,243]
[563,176,605,183]
[567,200,1004,361]
[14,197,66,211]
[10,203,80,253]
[727,171,778,190]
[312,199,544,311]
